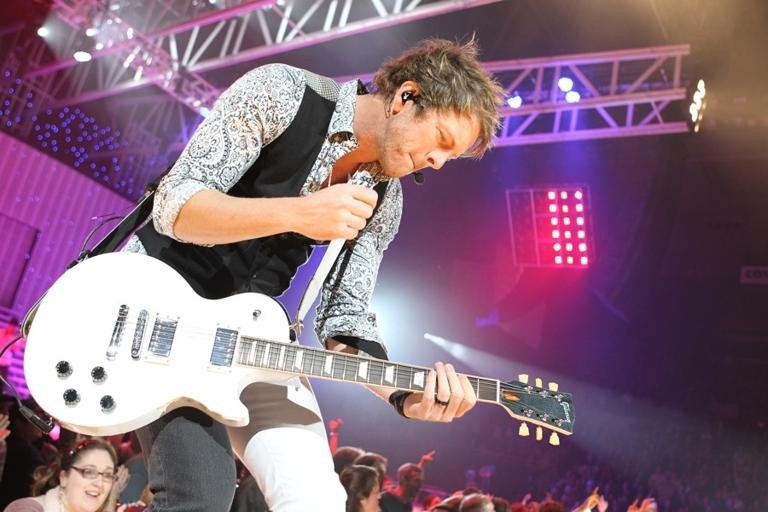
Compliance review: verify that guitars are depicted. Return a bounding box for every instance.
[23,252,576,447]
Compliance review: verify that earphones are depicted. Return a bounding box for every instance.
[402,91,411,101]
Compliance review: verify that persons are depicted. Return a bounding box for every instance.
[1,385,768,511]
[114,30,509,509]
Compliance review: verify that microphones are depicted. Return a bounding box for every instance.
[413,172,424,183]
[0,335,51,434]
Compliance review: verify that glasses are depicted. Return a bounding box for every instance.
[69,464,118,481]
